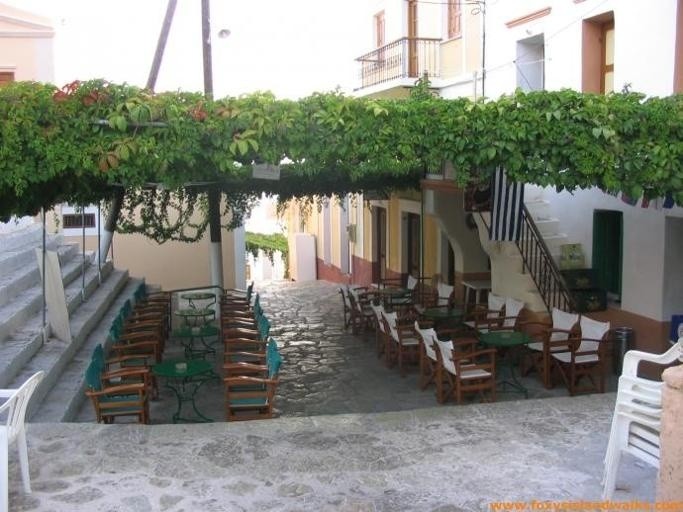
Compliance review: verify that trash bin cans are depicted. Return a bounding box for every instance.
[613,327,634,375]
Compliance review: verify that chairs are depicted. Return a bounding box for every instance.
[80,279,285,427]
[598,334,683,504]
[335,271,618,408]
[0,369,46,512]
